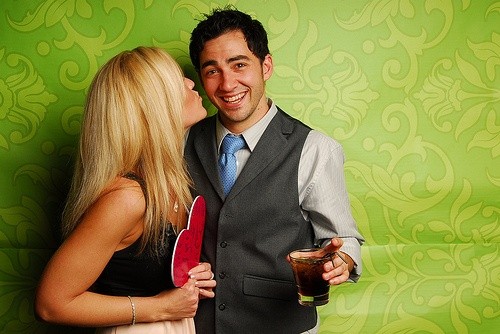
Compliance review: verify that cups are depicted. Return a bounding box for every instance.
[289,248,335,307]
